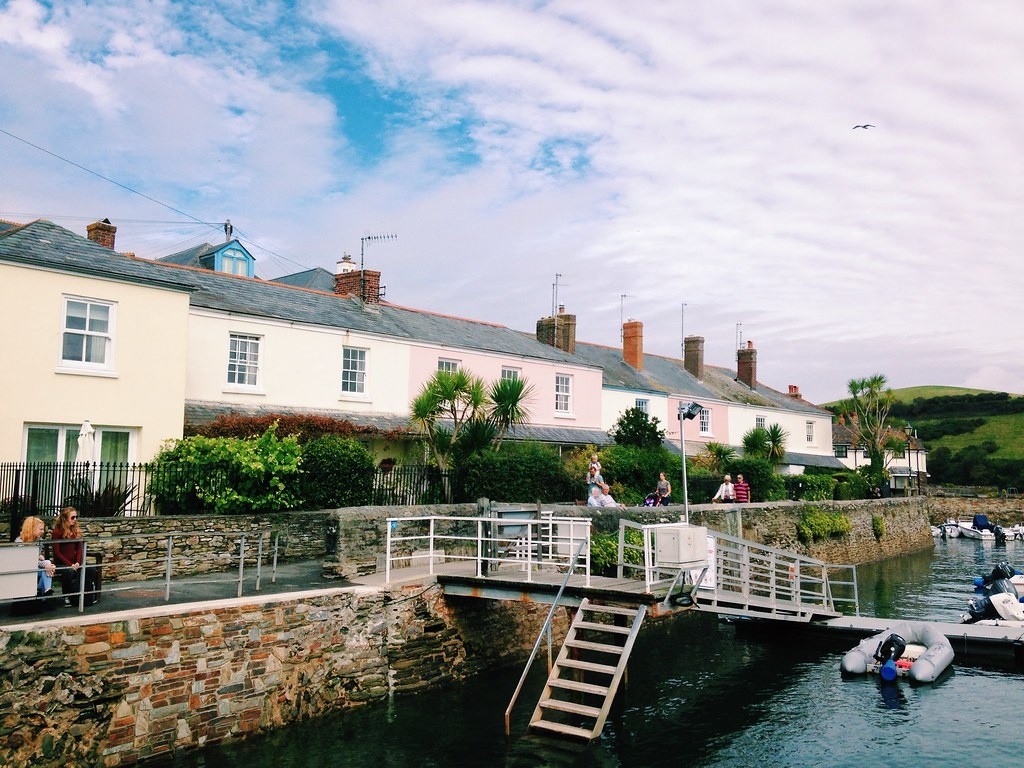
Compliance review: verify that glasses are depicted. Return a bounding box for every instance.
[68,515,79,520]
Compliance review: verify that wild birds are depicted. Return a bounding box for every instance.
[853,125,875,130]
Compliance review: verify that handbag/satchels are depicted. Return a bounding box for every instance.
[70,573,93,607]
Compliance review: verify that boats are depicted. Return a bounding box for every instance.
[1009,522,1024,540]
[930,517,962,538]
[972,561,1024,603]
[839,621,954,682]
[958,514,1015,540]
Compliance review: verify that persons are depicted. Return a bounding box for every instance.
[643,496,654,507]
[13,516,56,596]
[712,474,751,503]
[586,454,617,508]
[52,506,98,607]
[656,471,672,506]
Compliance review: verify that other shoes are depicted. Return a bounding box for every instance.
[44,588,54,597]
[63,596,72,607]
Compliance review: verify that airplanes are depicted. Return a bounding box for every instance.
[959,593,1024,626]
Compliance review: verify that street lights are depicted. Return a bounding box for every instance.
[904,422,913,496]
[678,400,704,527]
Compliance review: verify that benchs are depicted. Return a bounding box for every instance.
[575,498,588,506]
[38,545,103,601]
